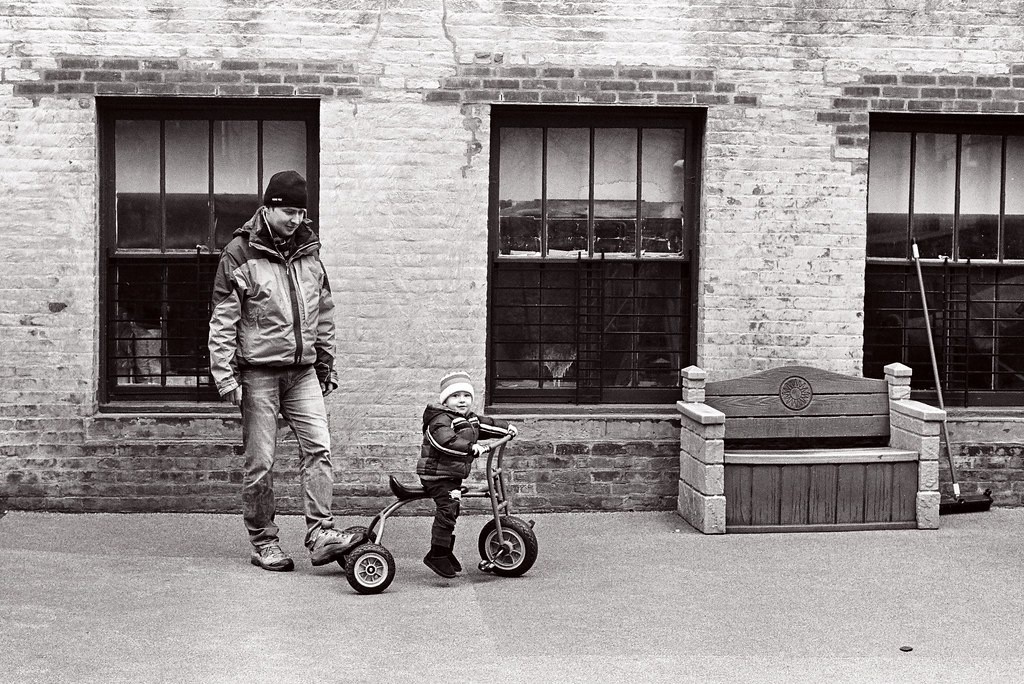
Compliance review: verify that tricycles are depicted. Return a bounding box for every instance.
[336,429,539,595]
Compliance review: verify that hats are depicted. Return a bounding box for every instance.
[263,170,308,209]
[439,369,475,406]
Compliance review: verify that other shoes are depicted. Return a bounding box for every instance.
[423,551,463,578]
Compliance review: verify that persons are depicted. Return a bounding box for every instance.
[208,171,368,573]
[416,369,518,579]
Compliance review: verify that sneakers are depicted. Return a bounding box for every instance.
[251,545,295,572]
[309,526,369,566]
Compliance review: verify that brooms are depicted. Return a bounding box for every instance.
[908,243,993,514]
